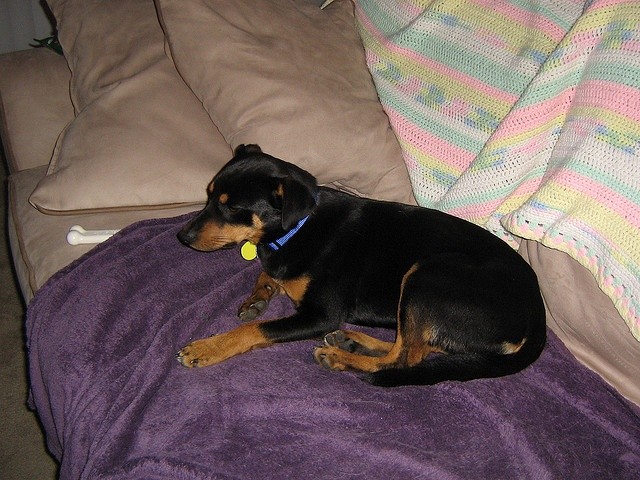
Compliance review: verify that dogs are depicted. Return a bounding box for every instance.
[174,141,546,388]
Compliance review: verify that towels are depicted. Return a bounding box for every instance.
[20,219,640,480]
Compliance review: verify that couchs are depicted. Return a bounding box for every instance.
[0,1,639,480]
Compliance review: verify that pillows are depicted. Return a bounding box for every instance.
[27,0,236,217]
[518,237,640,415]
[154,0,419,206]
